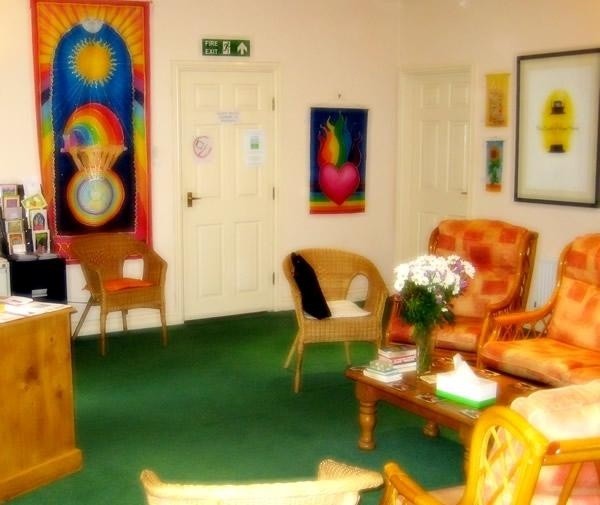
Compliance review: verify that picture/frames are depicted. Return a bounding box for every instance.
[514,48,600,208]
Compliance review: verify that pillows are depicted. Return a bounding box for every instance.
[291,251,331,317]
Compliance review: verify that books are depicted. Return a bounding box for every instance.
[363,344,417,383]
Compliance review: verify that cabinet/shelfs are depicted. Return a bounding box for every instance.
[11,258,66,303]
[0,295,83,502]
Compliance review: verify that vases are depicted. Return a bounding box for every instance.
[415,325,434,374]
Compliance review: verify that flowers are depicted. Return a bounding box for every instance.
[390,252,476,369]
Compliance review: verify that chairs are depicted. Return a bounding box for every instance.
[386,219,539,366]
[141,459,384,504]
[283,249,389,394]
[513,380,600,505]
[381,406,549,505]
[70,234,168,357]
[478,232,600,389]
